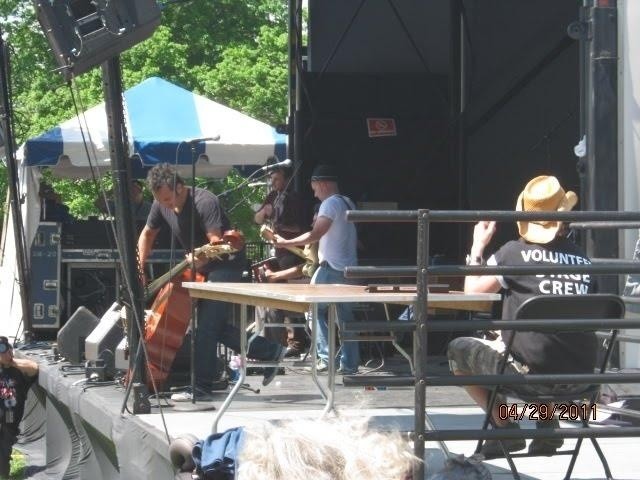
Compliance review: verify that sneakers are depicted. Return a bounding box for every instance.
[285,336,354,374]
[170,387,214,404]
[262,344,287,388]
[529,418,565,456]
[475,421,528,460]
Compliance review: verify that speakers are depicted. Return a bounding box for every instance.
[33,0,163,79]
[115,325,190,374]
[85,311,123,366]
[56,305,97,365]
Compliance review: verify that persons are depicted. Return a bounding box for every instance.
[255,165,304,270]
[93,179,152,221]
[274,165,359,375]
[447,174,598,457]
[235,389,426,480]
[137,161,287,391]
[0,336,39,480]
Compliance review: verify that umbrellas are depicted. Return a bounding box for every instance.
[24,76,285,304]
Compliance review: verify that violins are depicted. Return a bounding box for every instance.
[271,160,304,223]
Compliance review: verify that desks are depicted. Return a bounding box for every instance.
[176,278,500,465]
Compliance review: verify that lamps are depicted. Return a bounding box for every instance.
[82,300,126,370]
[114,332,130,373]
[56,304,101,369]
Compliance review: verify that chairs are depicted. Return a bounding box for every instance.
[457,290,627,479]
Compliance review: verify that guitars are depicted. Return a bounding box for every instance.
[259,224,318,278]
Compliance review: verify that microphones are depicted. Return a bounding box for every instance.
[248,181,273,189]
[184,134,221,145]
[264,158,292,170]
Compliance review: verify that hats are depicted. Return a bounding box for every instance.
[309,164,340,182]
[513,173,580,246]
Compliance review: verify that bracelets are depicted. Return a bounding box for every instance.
[465,254,482,266]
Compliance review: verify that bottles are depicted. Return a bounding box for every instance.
[227,355,240,384]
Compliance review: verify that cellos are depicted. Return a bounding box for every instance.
[129,231,245,395]
